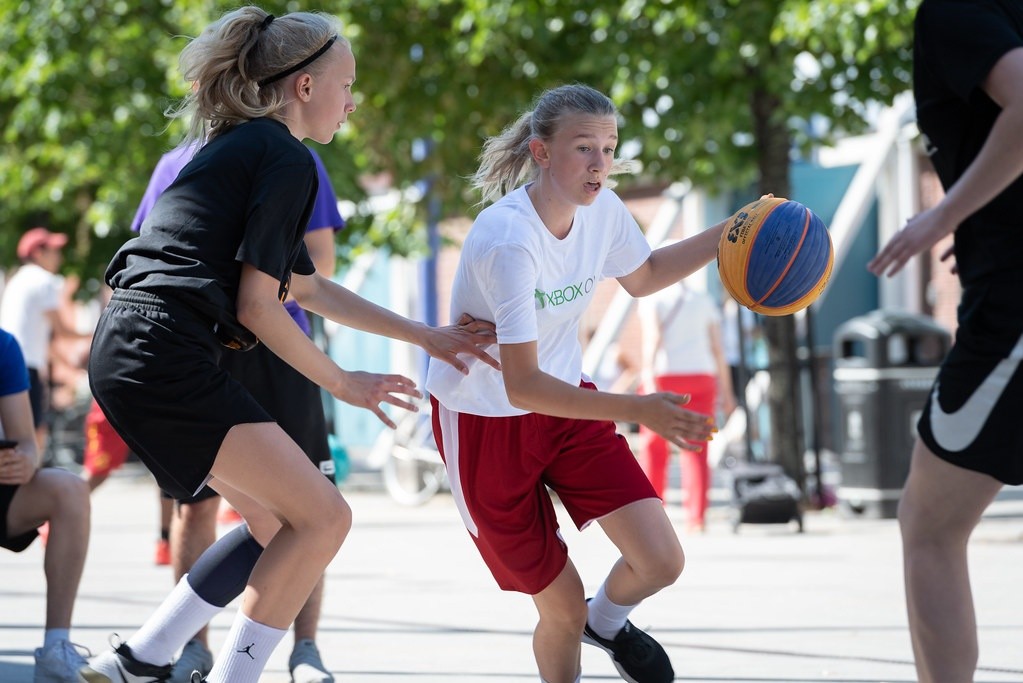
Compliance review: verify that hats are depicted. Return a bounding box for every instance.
[18,228,68,261]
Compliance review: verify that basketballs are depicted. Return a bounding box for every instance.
[716,198,833,317]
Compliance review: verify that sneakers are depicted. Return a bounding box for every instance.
[289,640,333,683]
[166,638,213,683]
[76,634,173,683]
[580,598,674,683]
[33,638,92,683]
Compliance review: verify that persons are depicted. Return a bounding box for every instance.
[0,229,94,456]
[0,329,91,682]
[81,7,502,682]
[37,398,172,565]
[426,85,776,682]
[864,0,1023,682]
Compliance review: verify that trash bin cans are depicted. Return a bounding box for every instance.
[831,305,954,521]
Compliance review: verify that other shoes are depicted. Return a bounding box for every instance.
[158,539,173,567]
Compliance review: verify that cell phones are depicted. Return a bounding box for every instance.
[0,439,19,451]
